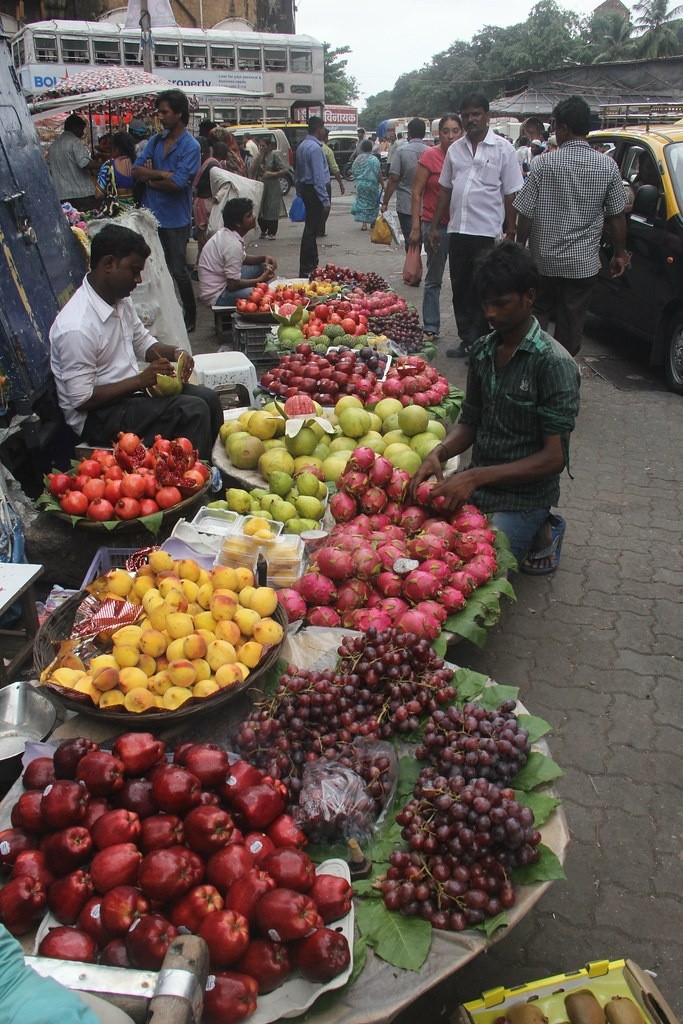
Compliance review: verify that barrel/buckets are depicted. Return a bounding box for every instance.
[0,680,58,795]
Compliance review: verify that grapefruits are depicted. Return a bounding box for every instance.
[270,302,309,343]
[139,352,183,397]
[219,394,448,480]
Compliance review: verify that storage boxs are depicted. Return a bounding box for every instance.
[79,545,144,592]
[448,958,682,1024]
[231,313,280,370]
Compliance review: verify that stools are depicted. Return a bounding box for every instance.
[211,305,237,346]
[191,352,261,421]
[0,562,45,689]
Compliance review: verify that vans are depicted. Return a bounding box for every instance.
[228,122,311,165]
[231,127,296,169]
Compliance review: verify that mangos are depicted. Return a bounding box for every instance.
[276,281,342,296]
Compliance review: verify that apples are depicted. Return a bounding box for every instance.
[0,732,354,1024]
[311,262,370,291]
[262,344,375,407]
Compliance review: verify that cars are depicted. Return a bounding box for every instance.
[396,131,434,147]
[325,135,362,182]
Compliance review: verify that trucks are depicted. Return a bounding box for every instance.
[379,116,431,144]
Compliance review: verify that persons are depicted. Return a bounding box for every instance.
[352,140,385,230]
[132,90,201,332]
[409,112,464,340]
[295,115,331,278]
[198,198,277,306]
[407,241,581,573]
[48,114,290,240]
[513,96,629,351]
[381,119,431,253]
[320,128,345,236]
[429,95,524,365]
[348,127,403,180]
[49,224,246,512]
[493,127,660,188]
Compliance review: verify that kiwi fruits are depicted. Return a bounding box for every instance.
[504,994,647,1024]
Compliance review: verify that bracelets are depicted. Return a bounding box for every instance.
[438,444,448,461]
[175,347,185,352]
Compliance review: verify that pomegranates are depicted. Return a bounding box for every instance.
[300,303,368,339]
[46,431,209,522]
[235,281,309,312]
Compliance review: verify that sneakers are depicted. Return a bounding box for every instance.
[446,342,473,358]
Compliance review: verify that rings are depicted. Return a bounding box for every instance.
[190,368,192,371]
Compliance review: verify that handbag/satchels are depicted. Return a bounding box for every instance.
[403,243,423,286]
[383,210,400,245]
[289,196,307,222]
[371,212,392,245]
[97,159,120,217]
[271,154,280,172]
[132,133,163,208]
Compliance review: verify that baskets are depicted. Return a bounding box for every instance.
[43,460,215,536]
[31,571,288,729]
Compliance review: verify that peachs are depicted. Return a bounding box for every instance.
[49,552,284,712]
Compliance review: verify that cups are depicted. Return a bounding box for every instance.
[299,530,328,548]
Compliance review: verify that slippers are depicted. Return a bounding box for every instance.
[269,234,275,240]
[521,515,565,575]
[259,232,267,239]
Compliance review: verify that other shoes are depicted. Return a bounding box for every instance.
[186,324,195,332]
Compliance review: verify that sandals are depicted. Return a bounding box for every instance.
[423,331,435,341]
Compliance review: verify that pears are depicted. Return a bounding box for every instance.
[204,469,328,537]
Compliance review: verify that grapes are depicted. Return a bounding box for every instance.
[231,626,542,930]
[363,271,391,294]
[367,305,423,354]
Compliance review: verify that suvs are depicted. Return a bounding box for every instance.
[578,102,683,393]
